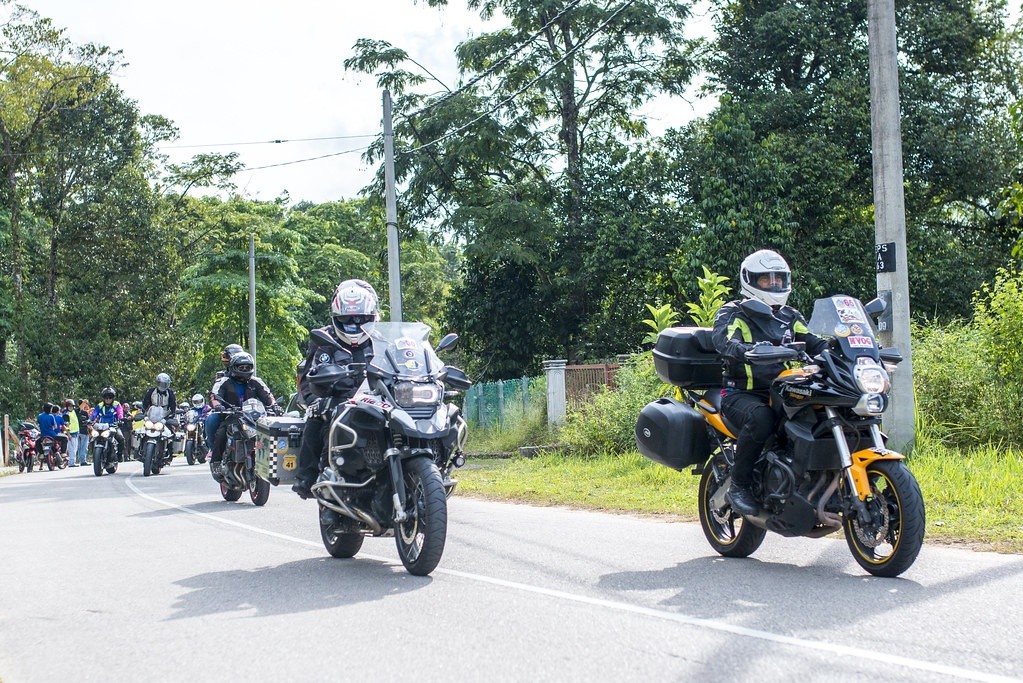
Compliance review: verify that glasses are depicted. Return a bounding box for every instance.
[336,315,370,324]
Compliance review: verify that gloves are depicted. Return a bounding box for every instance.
[306,397,323,418]
[215,405,226,416]
[747,341,773,351]
[273,406,285,416]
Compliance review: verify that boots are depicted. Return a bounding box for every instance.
[725,424,765,515]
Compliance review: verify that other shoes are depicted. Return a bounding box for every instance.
[61,453,68,457]
[69,464,79,467]
[204,448,213,462]
[39,468,43,470]
[118,453,122,462]
[292,477,309,495]
[210,460,224,480]
[39,454,45,459]
[81,462,91,466]
[319,508,341,526]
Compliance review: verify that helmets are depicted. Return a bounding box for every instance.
[156,373,170,391]
[228,351,255,381]
[331,279,378,347]
[101,387,115,404]
[66,399,75,406]
[191,394,205,409]
[221,343,244,367]
[179,402,190,413]
[740,249,792,311]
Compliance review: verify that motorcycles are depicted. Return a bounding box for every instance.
[210,394,286,506]
[13,409,210,477]
[634,293,926,578]
[254,322,472,577]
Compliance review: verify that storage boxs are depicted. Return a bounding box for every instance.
[634,397,710,472]
[253,417,305,486]
[653,326,723,389]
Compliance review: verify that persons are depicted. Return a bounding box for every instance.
[204,344,277,480]
[295,278,379,495]
[712,250,831,514]
[37,373,212,467]
